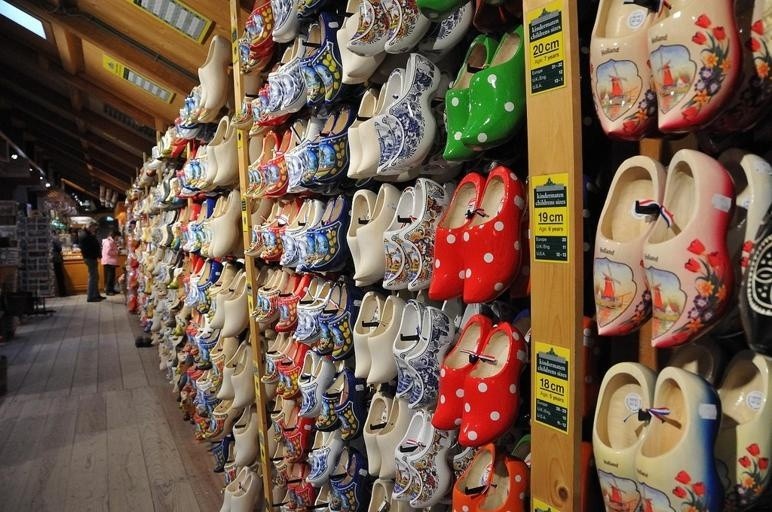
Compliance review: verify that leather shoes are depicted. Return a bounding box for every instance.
[107,291,120,295]
[88,296,106,302]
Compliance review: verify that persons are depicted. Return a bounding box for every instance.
[75,220,107,302]
[101,228,122,297]
[52,227,70,298]
